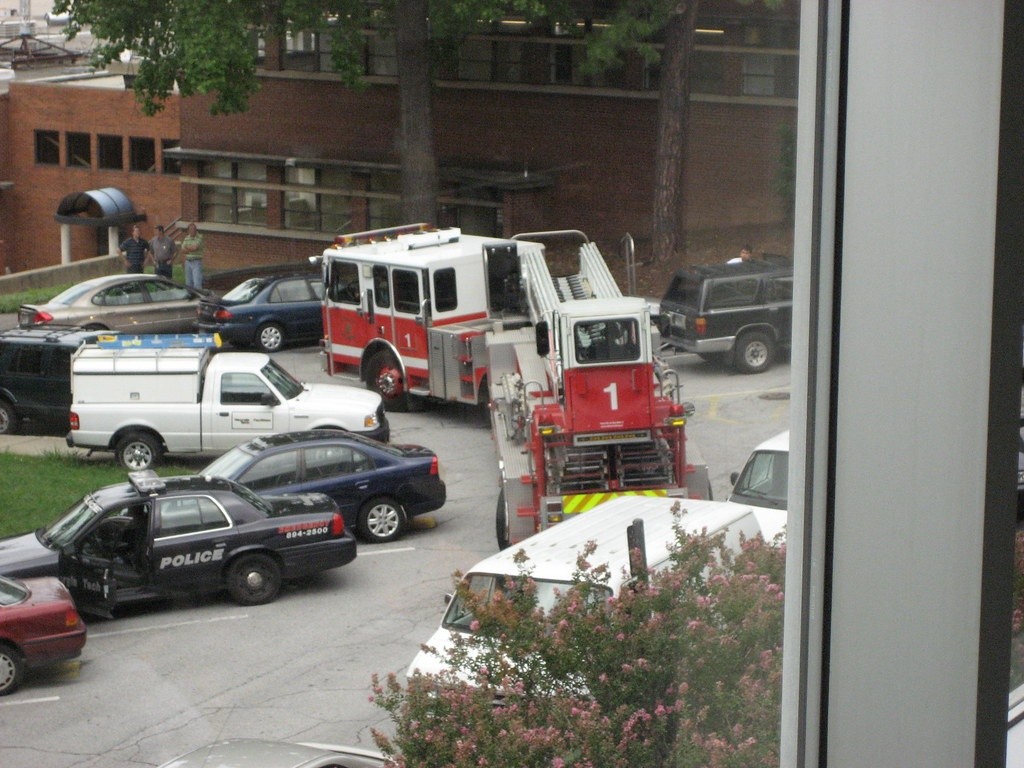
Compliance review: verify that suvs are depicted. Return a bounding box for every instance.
[0,322,118,435]
[657,252,796,375]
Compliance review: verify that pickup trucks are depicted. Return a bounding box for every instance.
[66,342,392,476]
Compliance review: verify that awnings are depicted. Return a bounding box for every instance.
[54,186,134,225]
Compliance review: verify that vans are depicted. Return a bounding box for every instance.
[408,495,792,719]
[727,430,791,546]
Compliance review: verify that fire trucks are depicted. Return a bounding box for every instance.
[308,220,715,555]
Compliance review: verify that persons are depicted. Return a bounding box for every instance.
[181,223,204,290]
[116,225,150,274]
[587,321,631,360]
[725,244,754,264]
[150,224,178,279]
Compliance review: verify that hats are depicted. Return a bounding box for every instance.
[600,323,621,338]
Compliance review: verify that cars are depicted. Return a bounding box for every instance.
[0,576,89,697]
[0,471,356,621]
[159,428,447,544]
[195,273,325,353]
[19,273,210,334]
